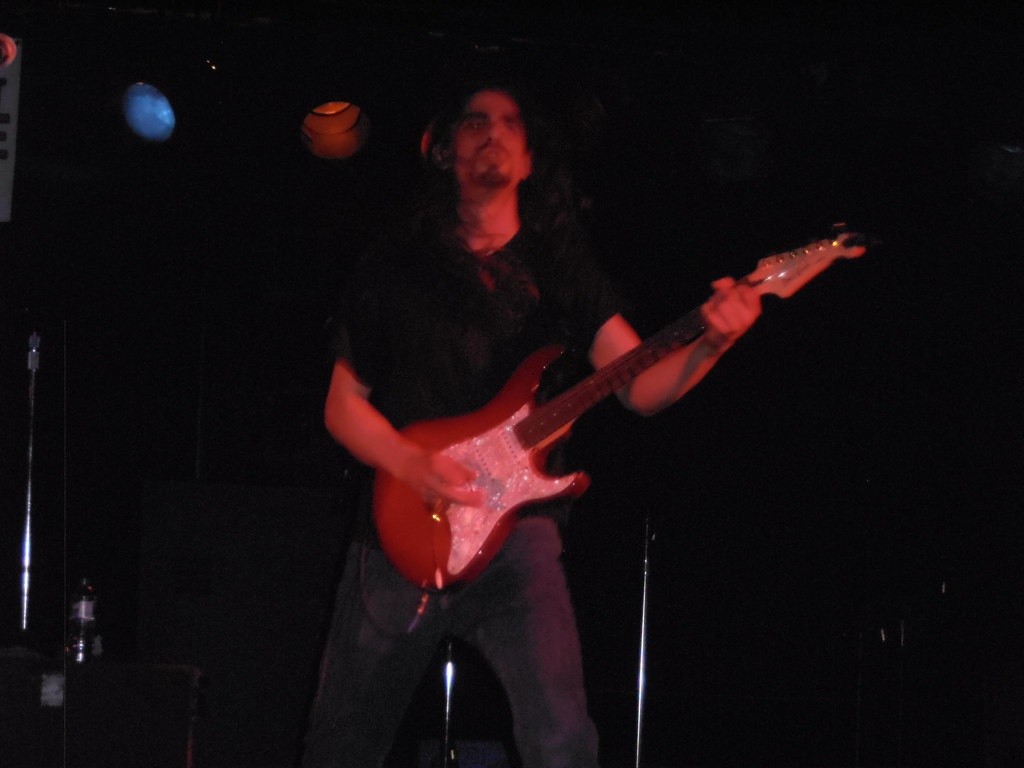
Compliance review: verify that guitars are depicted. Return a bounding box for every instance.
[373,234,863,589]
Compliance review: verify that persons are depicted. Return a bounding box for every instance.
[300,82,763,768]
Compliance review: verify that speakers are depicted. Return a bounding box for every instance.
[138,473,336,768]
[0,658,202,768]
